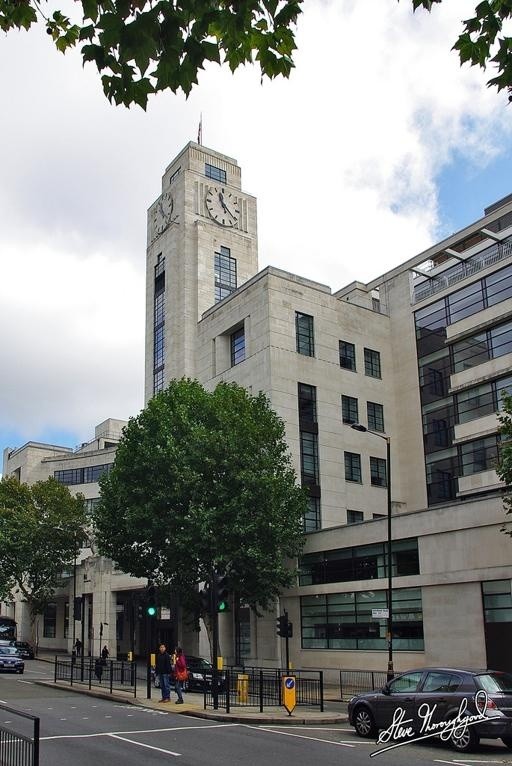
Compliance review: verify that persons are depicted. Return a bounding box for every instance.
[75,638,82,656]
[172,646,187,704]
[102,645,109,665]
[155,644,173,703]
[94,661,103,684]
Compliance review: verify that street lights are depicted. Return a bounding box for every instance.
[348,422,396,683]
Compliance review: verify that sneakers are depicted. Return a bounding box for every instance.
[158,698,171,703]
[175,696,183,704]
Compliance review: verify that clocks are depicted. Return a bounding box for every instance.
[152,192,173,233]
[205,186,242,228]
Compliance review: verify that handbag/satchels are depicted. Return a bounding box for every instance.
[175,668,188,681]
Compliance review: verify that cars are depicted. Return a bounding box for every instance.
[0,616,35,674]
[348,668,511,749]
[152,655,223,694]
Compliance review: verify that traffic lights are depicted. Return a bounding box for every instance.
[218,577,228,612]
[148,586,157,616]
[275,613,288,638]
